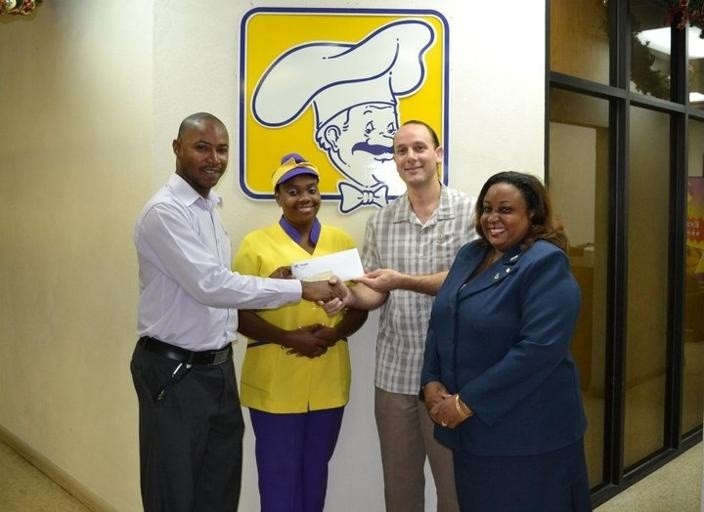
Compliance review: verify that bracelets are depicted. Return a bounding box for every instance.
[455,394,469,419]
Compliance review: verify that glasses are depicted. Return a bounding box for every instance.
[150,362,193,402]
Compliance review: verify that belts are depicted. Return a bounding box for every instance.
[138,335,233,365]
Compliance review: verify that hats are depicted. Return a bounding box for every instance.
[272,153,320,195]
[251,19,436,129]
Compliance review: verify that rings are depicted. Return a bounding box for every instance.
[441,421,447,427]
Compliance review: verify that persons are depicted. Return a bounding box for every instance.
[315,120,478,512]
[231,152,369,512]
[418,170,592,511]
[130,113,347,512]
